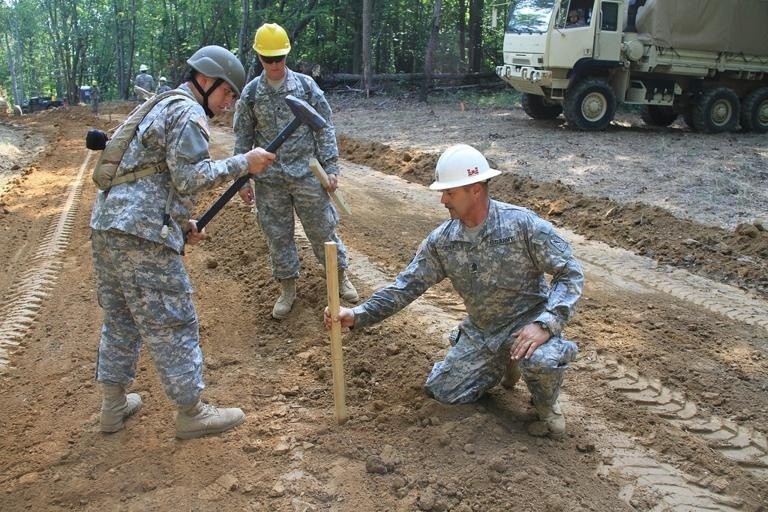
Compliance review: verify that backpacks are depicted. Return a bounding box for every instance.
[92,84,197,192]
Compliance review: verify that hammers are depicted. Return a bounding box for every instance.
[183,93,328,243]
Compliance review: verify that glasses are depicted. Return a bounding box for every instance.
[260,54,286,64]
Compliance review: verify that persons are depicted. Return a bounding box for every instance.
[89,79,103,114]
[89,45,277,441]
[231,20,361,321]
[156,78,172,96]
[322,141,587,438]
[133,63,154,101]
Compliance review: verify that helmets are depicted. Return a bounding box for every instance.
[159,76,166,81]
[569,10,578,17]
[428,143,502,190]
[139,64,149,70]
[252,22,291,57]
[186,44,246,99]
[92,80,97,86]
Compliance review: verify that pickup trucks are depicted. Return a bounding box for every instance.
[21,97,64,114]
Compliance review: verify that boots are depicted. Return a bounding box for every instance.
[272,278,296,319]
[533,393,566,438]
[99,381,144,433]
[501,358,521,387]
[175,397,246,440]
[338,269,359,303]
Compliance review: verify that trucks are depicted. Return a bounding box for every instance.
[494,0,768,133]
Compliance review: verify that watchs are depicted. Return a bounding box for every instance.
[535,320,551,333]
